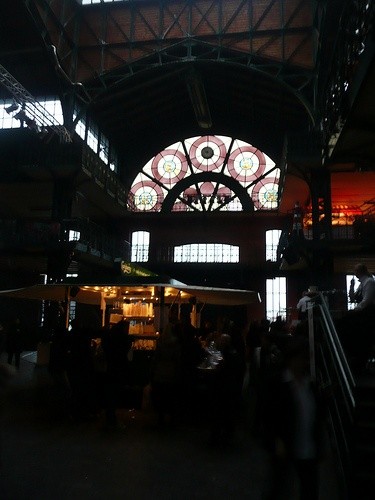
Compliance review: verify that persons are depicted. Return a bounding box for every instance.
[4,316,25,367]
[349,264,375,371]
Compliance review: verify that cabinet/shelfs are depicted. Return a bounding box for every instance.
[123,298,159,351]
